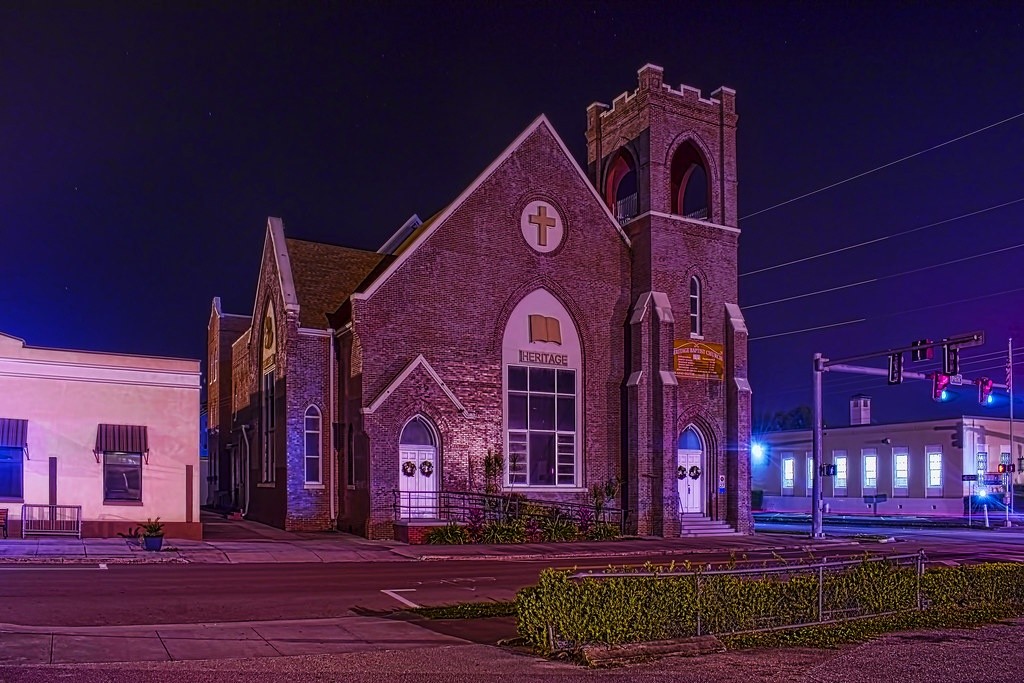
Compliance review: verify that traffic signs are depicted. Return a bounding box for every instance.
[962,475,977,481]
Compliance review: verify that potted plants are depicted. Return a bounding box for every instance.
[137,517,165,551]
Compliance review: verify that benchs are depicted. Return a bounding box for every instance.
[0,508,8,539]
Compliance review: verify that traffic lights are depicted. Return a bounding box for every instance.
[978,377,993,405]
[931,371,947,400]
[998,464,1006,473]
[825,464,837,475]
[1007,464,1015,472]
